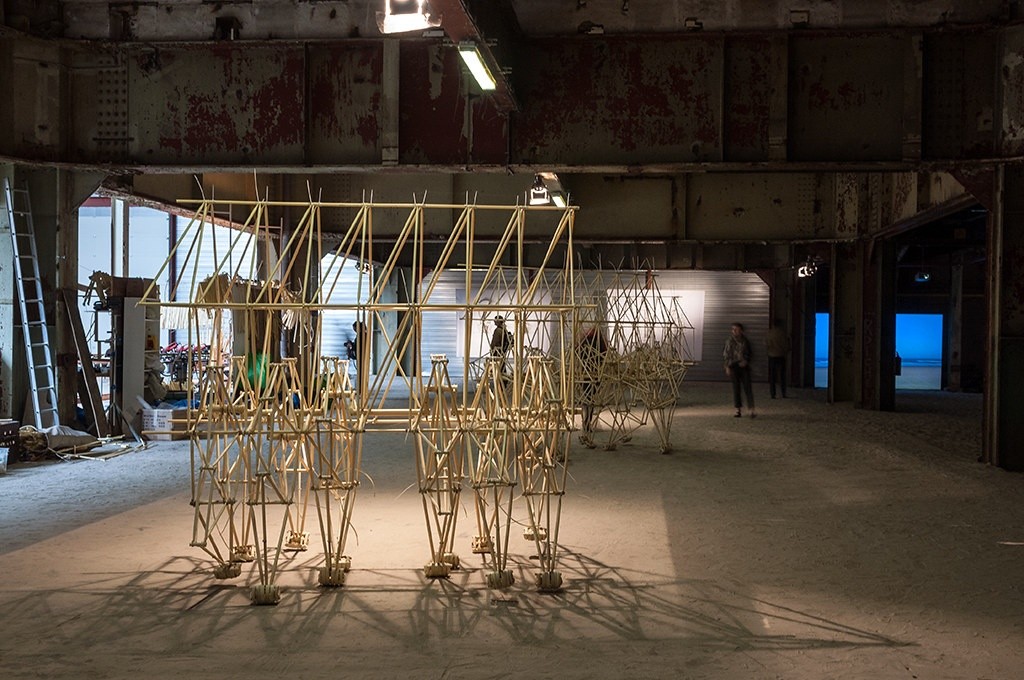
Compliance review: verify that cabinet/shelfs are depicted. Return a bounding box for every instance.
[120,296,162,443]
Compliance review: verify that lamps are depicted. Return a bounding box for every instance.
[374,0,443,35]
[457,39,499,92]
[527,171,569,210]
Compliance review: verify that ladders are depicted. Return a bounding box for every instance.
[5,176,60,429]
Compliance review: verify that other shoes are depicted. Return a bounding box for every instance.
[734,412,741,417]
[751,413,756,418]
[771,396,776,399]
[782,395,787,398]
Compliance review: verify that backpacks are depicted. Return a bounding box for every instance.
[499,327,514,353]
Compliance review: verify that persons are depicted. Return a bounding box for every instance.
[721,323,757,419]
[894,348,902,376]
[950,351,966,392]
[490,315,514,389]
[763,320,789,399]
[345,320,368,395]
[574,313,608,433]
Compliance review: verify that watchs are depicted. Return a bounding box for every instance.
[349,346,353,350]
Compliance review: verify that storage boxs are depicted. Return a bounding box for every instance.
[141,406,195,442]
